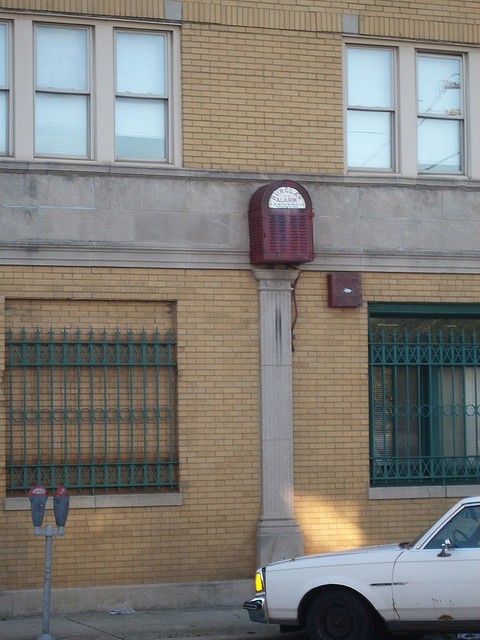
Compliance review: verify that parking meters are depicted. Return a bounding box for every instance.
[27,484,71,639]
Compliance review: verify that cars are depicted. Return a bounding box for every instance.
[244,495,479,639]
